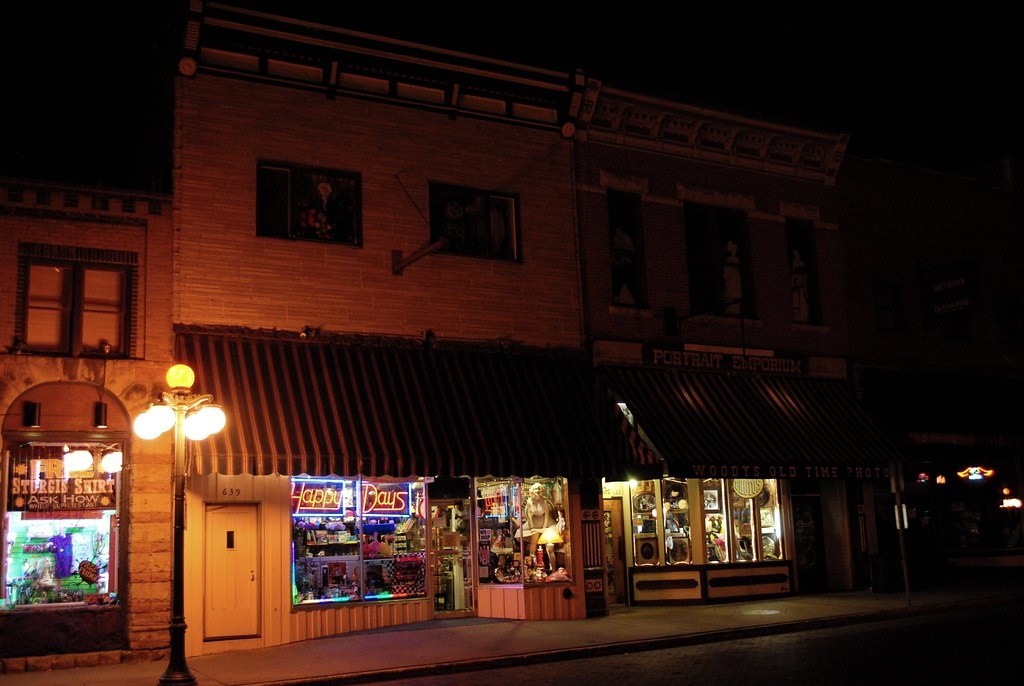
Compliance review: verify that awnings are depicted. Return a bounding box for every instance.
[173,321,629,483]
[597,363,929,482]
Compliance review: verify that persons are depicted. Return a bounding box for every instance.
[556,509,565,534]
[514,482,557,573]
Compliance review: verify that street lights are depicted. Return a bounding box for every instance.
[131,363,228,686]
